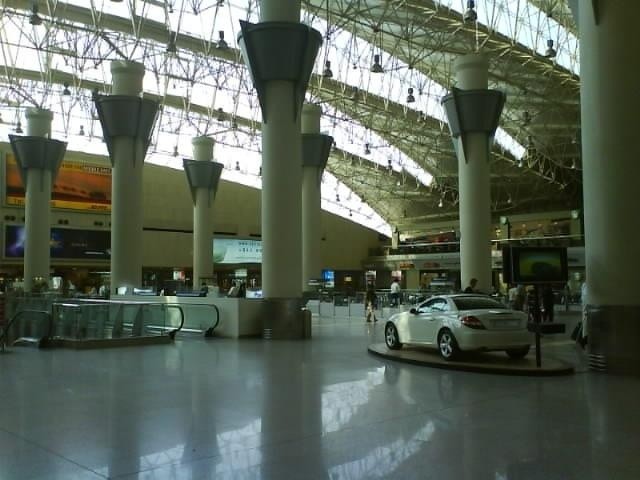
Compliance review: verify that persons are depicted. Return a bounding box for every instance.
[199,281,209,297]
[389,278,401,308]
[508,284,554,324]
[464,278,480,294]
[363,283,379,323]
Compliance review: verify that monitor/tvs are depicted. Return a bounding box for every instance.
[172,270,185,281]
[322,271,334,280]
[234,268,248,278]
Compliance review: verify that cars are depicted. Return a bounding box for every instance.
[551,284,578,305]
[385,290,536,362]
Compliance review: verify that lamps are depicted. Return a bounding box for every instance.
[544,39,556,58]
[321,60,333,78]
[370,54,383,73]
[463,1,478,22]
[165,30,177,52]
[215,30,228,49]
[29,2,42,25]
[16,84,580,217]
